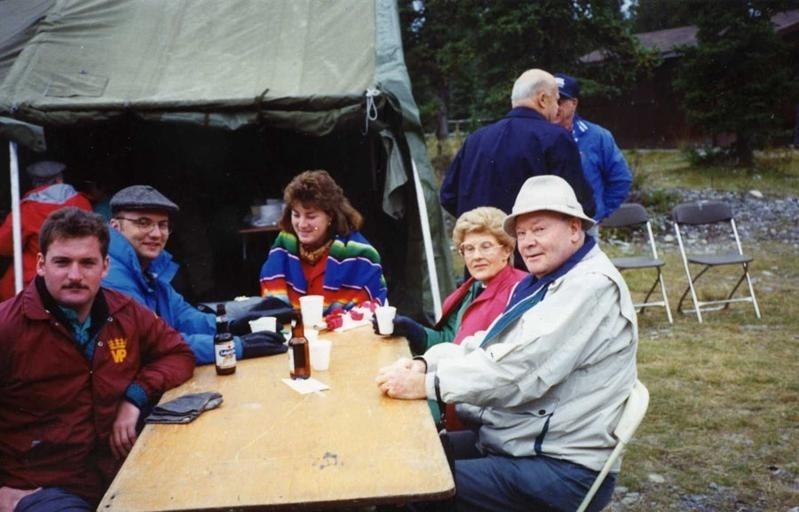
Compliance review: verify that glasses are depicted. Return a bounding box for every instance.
[116,216,174,237]
[458,241,506,257]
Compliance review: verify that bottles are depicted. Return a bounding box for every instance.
[212,303,236,376]
[288,313,310,381]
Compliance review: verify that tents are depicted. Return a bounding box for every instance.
[0,1,450,330]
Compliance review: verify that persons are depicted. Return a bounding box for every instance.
[443,69,596,282]
[96,184,292,366]
[1,207,193,510]
[260,171,388,317]
[0,161,94,305]
[550,73,634,249]
[377,176,637,512]
[83,170,120,225]
[373,205,528,431]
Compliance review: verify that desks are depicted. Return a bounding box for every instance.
[94,302,459,510]
[232,221,284,255]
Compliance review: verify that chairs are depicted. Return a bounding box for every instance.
[571,378,652,509]
[593,202,675,325]
[671,198,765,322]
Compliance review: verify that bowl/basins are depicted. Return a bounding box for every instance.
[258,205,281,221]
[251,206,259,216]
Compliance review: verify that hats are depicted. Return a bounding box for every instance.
[109,183,181,215]
[27,159,66,179]
[501,174,598,240]
[552,73,581,101]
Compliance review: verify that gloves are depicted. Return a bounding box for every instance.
[369,309,428,347]
[228,305,295,334]
[237,325,290,360]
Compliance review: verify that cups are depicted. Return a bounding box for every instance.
[299,295,323,327]
[250,316,277,336]
[305,329,321,339]
[374,306,396,336]
[310,339,330,372]
